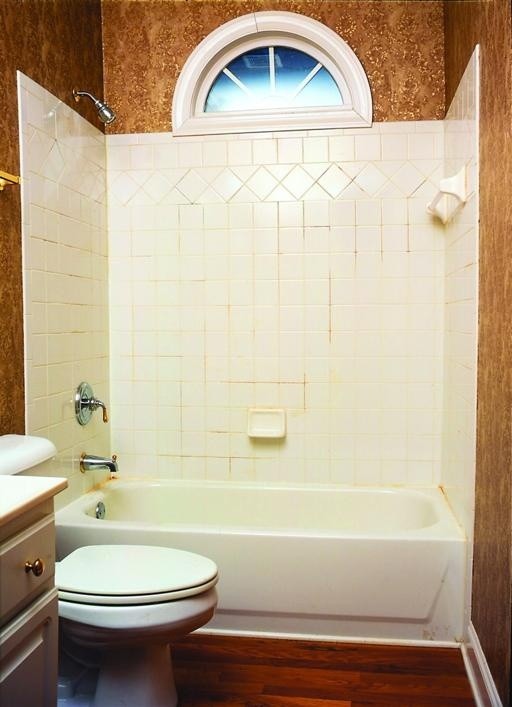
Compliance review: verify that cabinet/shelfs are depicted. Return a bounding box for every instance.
[0,497,59,707]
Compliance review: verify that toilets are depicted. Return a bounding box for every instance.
[0,432,219,707]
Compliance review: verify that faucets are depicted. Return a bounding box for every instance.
[77,451,121,473]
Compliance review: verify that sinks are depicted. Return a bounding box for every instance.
[56,477,468,651]
